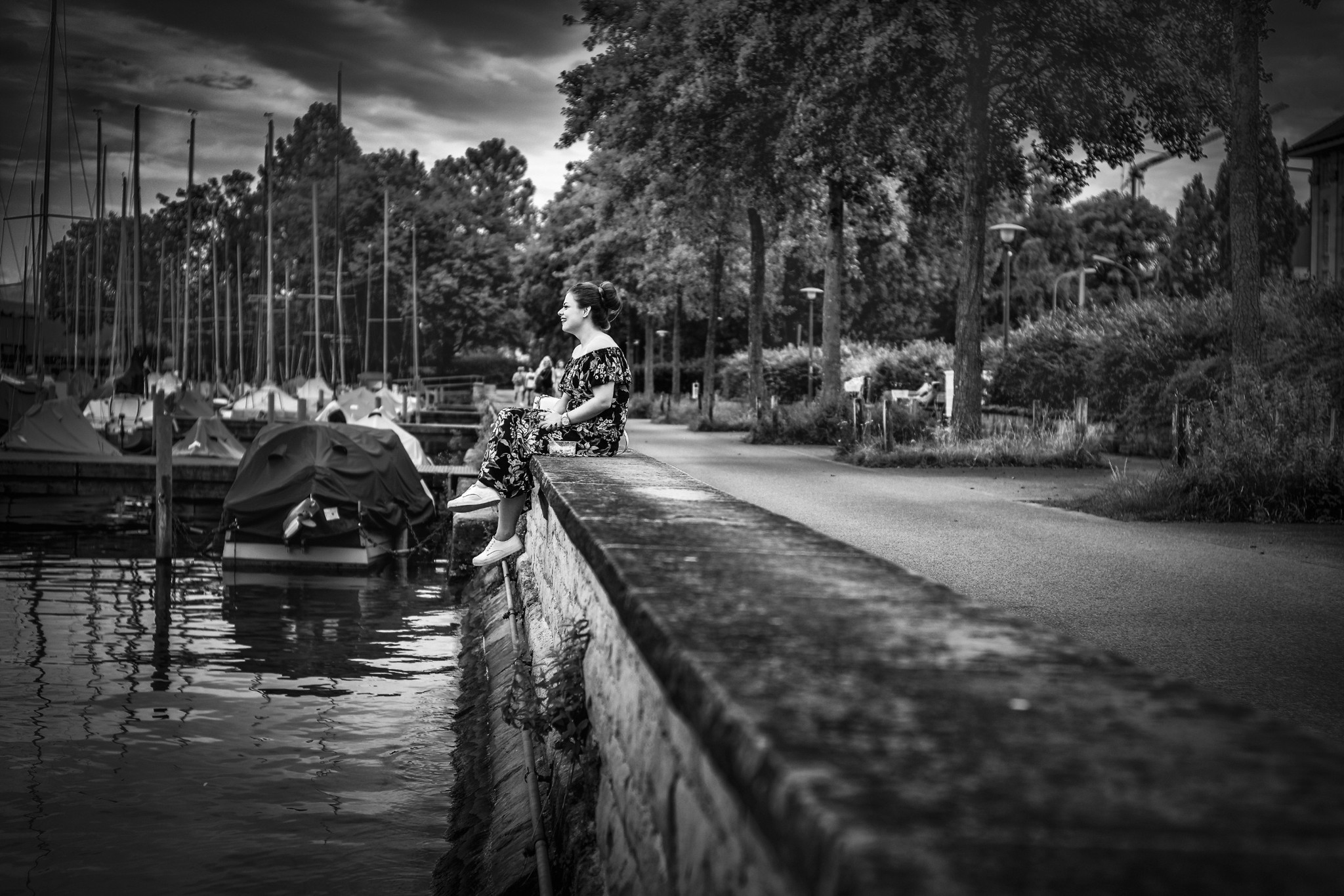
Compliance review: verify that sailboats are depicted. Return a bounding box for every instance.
[0,1,442,466]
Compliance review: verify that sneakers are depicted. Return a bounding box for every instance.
[472,531,523,566]
[447,484,500,513]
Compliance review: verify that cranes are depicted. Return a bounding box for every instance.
[1120,102,1290,198]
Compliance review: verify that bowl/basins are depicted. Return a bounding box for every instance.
[549,441,576,457]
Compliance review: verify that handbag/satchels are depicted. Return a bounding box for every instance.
[533,395,562,410]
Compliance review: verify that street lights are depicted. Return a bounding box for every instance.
[989,222,1027,357]
[1094,255,1142,301]
[1053,267,1096,319]
[655,330,668,364]
[800,287,823,401]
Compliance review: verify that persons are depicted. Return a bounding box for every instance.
[445,281,632,566]
[513,355,565,406]
[905,371,934,407]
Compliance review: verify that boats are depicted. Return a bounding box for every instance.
[221,422,438,574]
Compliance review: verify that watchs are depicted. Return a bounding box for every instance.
[560,412,571,428]
